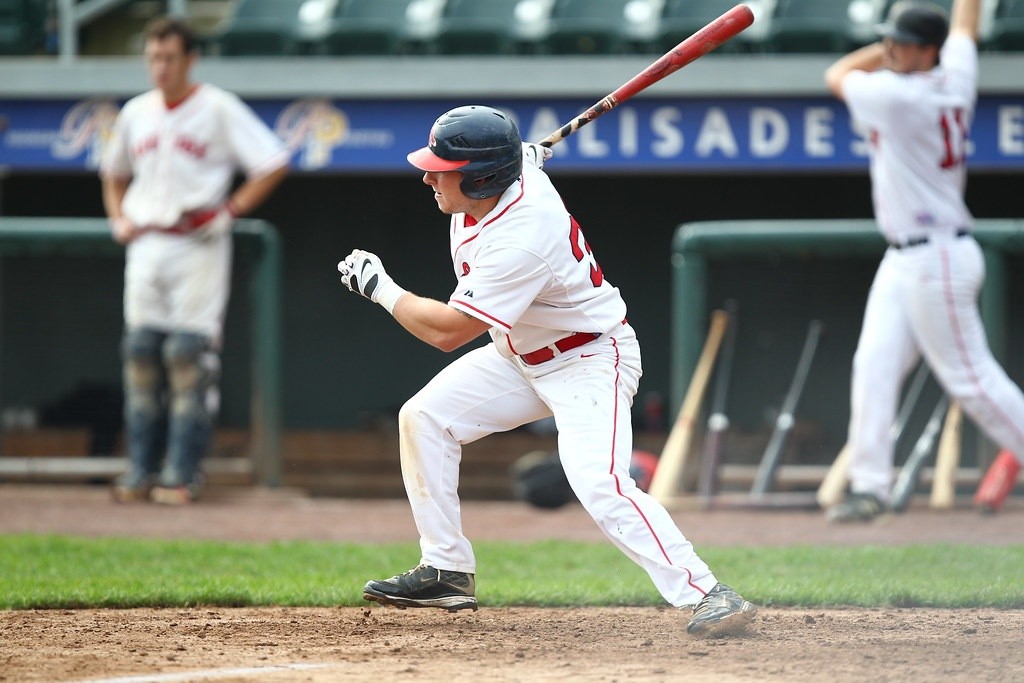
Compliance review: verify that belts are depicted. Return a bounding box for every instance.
[892,228,969,250]
[520,331,599,365]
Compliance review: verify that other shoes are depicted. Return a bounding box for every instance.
[826,493,887,524]
[113,476,204,503]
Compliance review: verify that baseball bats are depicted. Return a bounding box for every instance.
[540,3,757,151]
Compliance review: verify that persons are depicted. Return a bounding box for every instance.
[97,21,289,505]
[338,107,758,637]
[824,0,1024,523]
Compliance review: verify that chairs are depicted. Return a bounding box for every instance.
[208,1,1023,55]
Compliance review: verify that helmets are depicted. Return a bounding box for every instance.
[406,103,524,200]
[872,8,950,46]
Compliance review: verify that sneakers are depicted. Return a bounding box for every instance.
[362,565,479,609]
[685,583,759,637]
